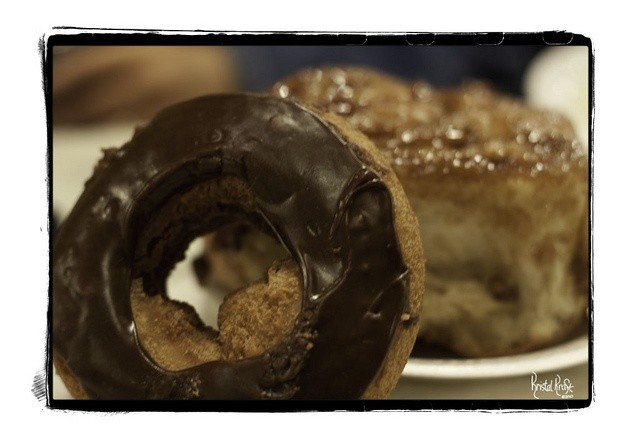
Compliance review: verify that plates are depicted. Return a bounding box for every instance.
[163,234,590,380]
